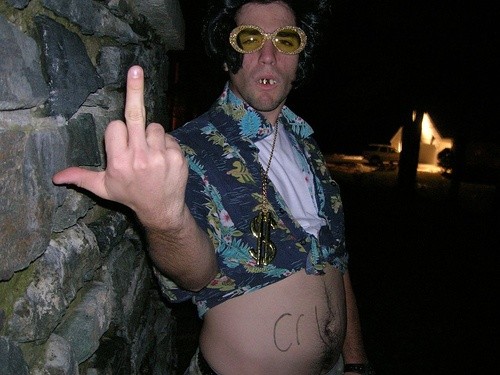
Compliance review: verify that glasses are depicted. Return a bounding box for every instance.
[228,25,307,55]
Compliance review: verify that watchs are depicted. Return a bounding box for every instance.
[343,361,372,375]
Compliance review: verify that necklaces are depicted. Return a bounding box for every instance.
[248,118,280,266]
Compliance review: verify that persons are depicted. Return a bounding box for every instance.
[50,0,372,375]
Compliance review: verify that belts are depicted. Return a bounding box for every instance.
[197,347,218,375]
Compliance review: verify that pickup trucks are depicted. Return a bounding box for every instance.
[368,145,401,166]
